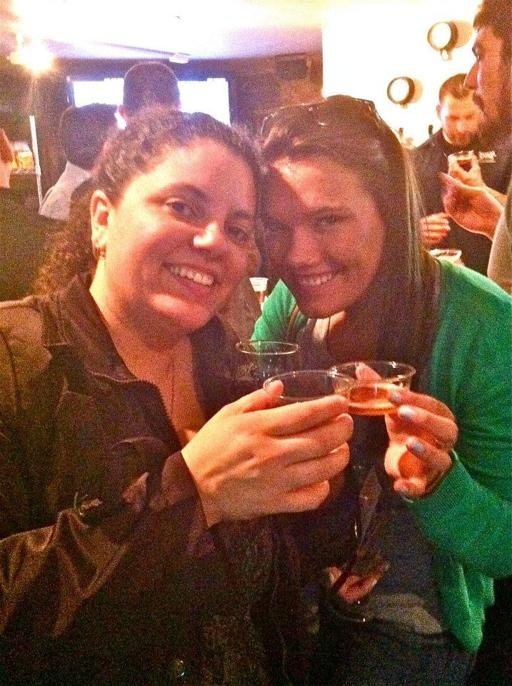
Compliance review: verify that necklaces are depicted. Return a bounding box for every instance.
[166,351,183,423]
[323,315,376,361]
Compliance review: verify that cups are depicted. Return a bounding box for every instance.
[450,147,474,168]
[234,339,300,397]
[263,360,416,467]
[431,249,463,267]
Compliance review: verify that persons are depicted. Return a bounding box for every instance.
[72,62,184,204]
[1,98,360,686]
[398,69,507,283]
[34,102,120,227]
[1,105,71,303]
[433,0,512,296]
[248,92,512,685]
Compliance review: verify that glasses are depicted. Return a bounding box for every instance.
[261,94,381,136]
